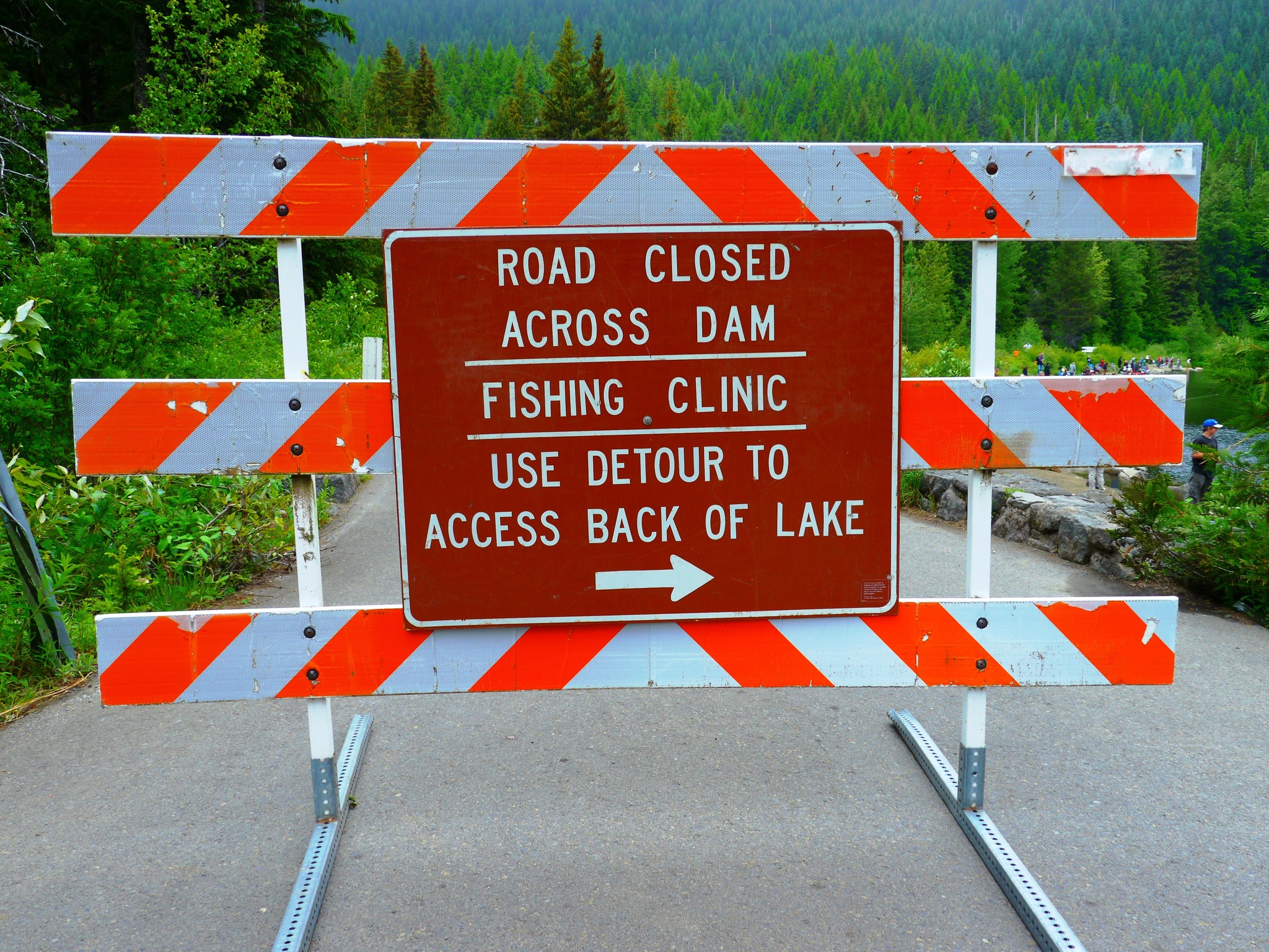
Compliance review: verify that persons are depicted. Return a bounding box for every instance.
[1118,354,1181,375]
[1034,352,1052,376]
[1088,467,1106,494]
[994,366,1000,377]
[1082,355,1109,375]
[1023,342,1032,352]
[1184,419,1224,511]
[1187,358,1191,368]
[1059,362,1077,376]
[1013,348,1019,358]
[1022,366,1028,376]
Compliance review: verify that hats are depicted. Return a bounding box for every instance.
[1202,419,1224,428]
[1062,366,1066,368]
[1088,355,1090,357]
